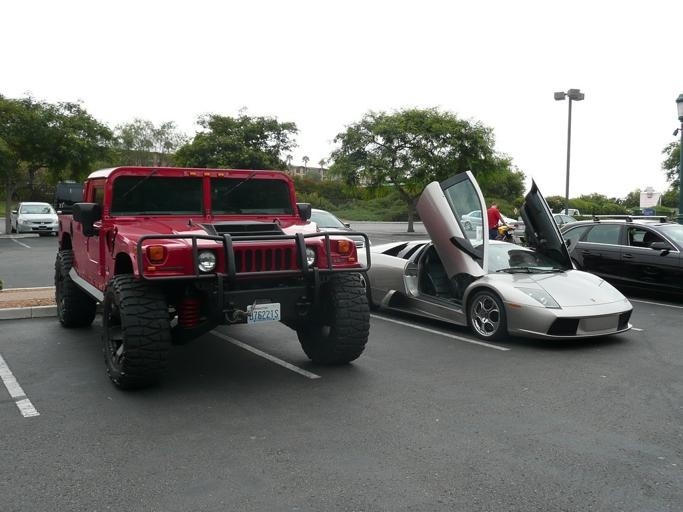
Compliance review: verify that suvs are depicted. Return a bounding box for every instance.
[54,165,371,390]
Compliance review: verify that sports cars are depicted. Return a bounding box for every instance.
[353,169,634,341]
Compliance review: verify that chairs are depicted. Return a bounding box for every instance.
[422,247,452,298]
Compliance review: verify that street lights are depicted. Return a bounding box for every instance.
[554,88,585,215]
[676,93,683,225]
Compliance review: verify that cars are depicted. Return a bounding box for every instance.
[10,202,59,236]
[557,169,683,341]
[460,210,517,232]
[307,208,372,248]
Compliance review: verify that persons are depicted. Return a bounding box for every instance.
[487,202,506,239]
